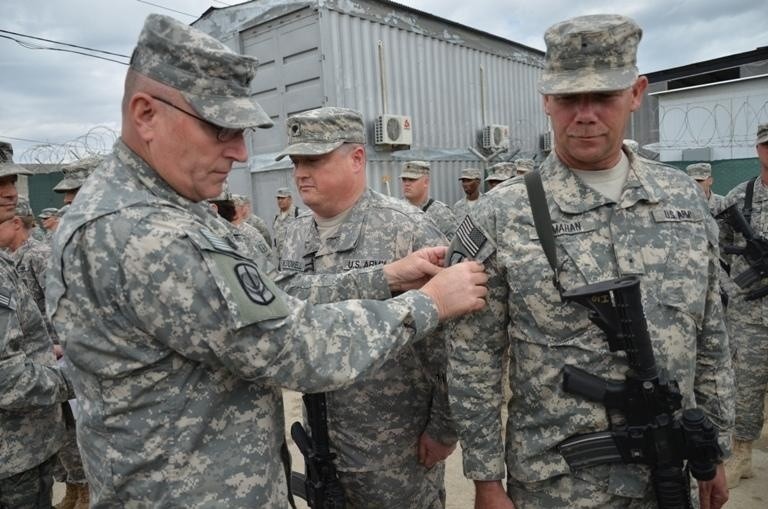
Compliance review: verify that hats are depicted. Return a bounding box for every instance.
[536,15,641,94]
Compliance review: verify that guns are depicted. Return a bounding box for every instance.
[290,393,348,509]
[713,202,767,302]
[558,277,723,507]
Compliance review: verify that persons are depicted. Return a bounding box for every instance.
[0,140,108,509]
[685,162,730,220]
[451,168,490,221]
[43,12,492,509]
[714,119,767,494]
[486,159,513,192]
[205,183,306,247]
[508,157,535,177]
[397,158,461,242]
[446,10,741,509]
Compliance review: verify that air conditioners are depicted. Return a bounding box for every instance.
[374,114,412,147]
[482,124,509,151]
[543,131,554,152]
